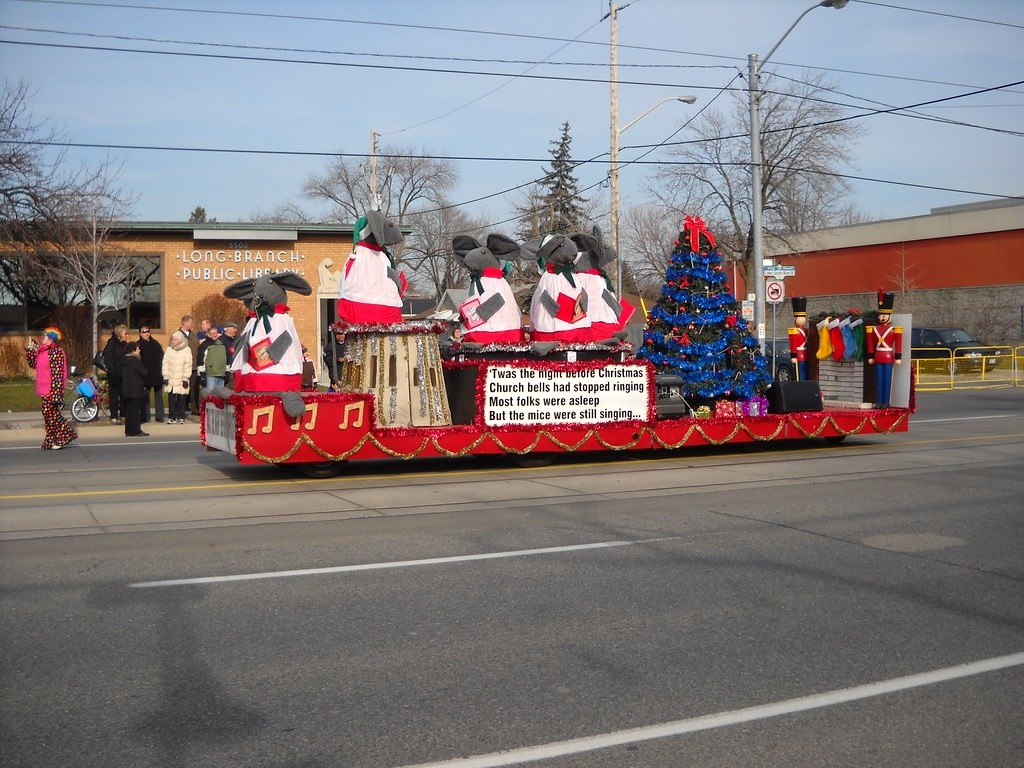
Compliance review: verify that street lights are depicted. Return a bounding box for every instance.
[748,0,851,356]
[610,95,698,297]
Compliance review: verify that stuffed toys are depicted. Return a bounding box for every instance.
[452,233,526,349]
[337,210,404,324]
[529,233,620,355]
[575,225,629,339]
[201,272,313,417]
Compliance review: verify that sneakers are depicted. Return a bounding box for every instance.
[167,419,177,424]
[126,432,149,436]
[179,419,184,424]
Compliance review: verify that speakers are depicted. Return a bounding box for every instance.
[767,380,823,414]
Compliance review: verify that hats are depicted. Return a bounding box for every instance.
[226,322,239,330]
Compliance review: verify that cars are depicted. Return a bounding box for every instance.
[911,326,1003,374]
[764,338,795,382]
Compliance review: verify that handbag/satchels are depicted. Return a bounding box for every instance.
[301,357,313,386]
[91,340,115,372]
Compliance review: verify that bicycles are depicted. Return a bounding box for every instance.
[61,364,120,422]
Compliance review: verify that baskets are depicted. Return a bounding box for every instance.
[77,376,97,398]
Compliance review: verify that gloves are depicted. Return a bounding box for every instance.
[200,372,206,387]
[225,371,230,384]
[183,381,188,388]
[162,379,168,386]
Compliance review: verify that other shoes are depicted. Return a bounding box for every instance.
[52,445,63,449]
[185,408,192,413]
[139,419,151,423]
[155,418,164,422]
[110,418,121,424]
[120,417,126,423]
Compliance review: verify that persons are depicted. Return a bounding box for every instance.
[197,327,230,388]
[104,325,128,424]
[865,287,903,409]
[162,331,193,424]
[196,319,223,342]
[26,327,78,450]
[136,325,165,423]
[449,328,464,343]
[219,321,239,365]
[324,334,345,389]
[121,342,150,436]
[170,315,200,416]
[524,326,529,340]
[788,297,808,381]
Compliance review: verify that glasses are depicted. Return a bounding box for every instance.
[141,331,150,333]
[124,333,129,335]
